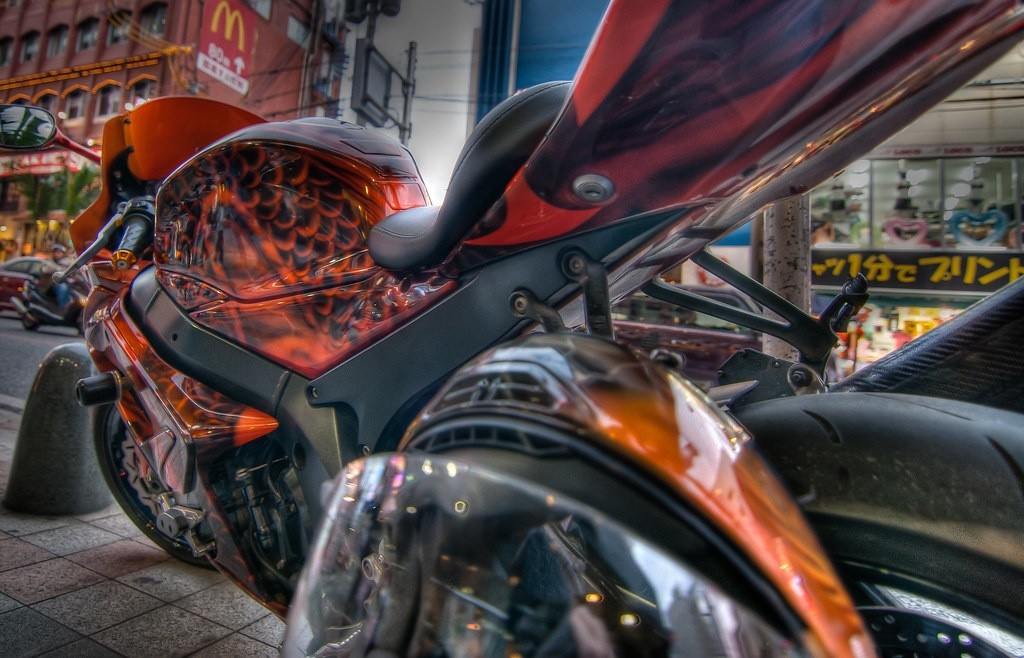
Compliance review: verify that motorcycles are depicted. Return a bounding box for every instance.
[10,260,98,337]
[0,1,1024,656]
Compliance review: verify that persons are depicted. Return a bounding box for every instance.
[35,243,71,309]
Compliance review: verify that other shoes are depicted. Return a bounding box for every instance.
[62,308,70,316]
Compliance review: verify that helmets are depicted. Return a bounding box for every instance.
[51,243,66,252]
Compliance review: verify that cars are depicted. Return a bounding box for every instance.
[0,255,58,307]
[613,287,763,387]
